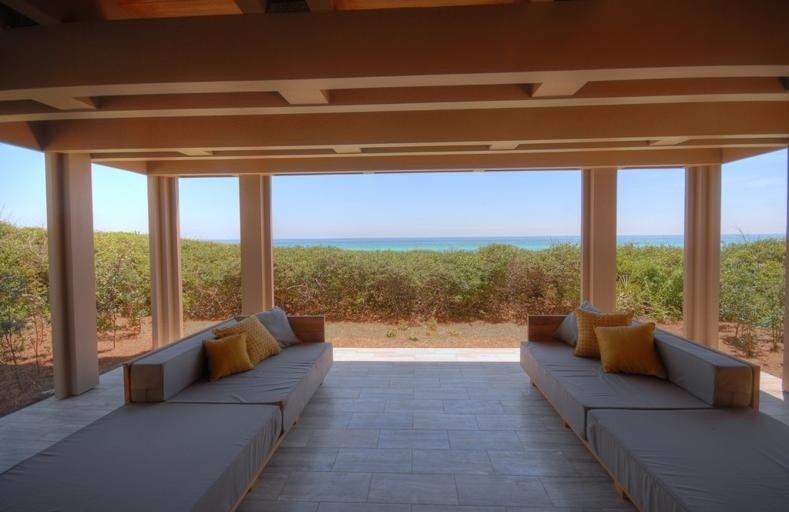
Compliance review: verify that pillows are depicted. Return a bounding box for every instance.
[594,322,666,378]
[254,307,301,346]
[212,316,282,364]
[197,332,254,382]
[553,301,594,346]
[571,307,633,359]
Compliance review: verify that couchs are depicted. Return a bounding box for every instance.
[128,305,334,435]
[516,300,778,441]
[0,404,282,511]
[586,409,785,510]
